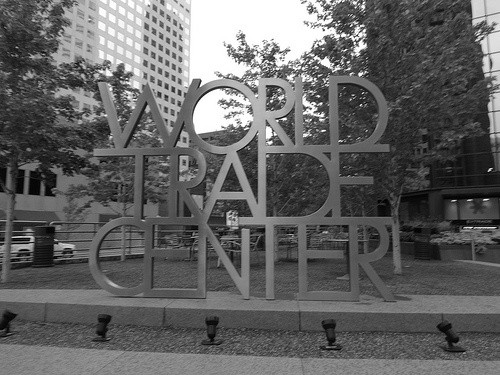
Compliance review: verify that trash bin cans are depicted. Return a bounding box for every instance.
[31,225,57,268]
[411,225,434,261]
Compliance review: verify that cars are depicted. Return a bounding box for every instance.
[0,236,75,264]
